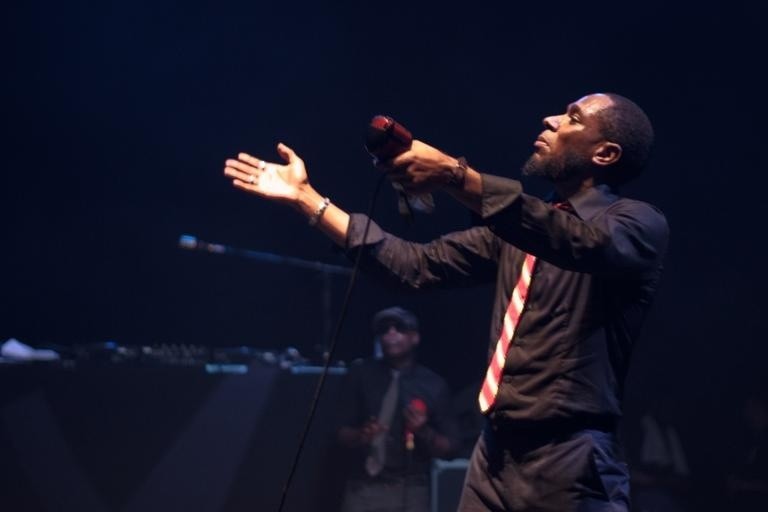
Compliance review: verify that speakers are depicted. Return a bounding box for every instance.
[431,458,471,512]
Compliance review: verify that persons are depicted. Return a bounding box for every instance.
[221,88,672,511]
[339,307,463,511]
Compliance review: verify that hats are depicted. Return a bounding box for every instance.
[374,305,418,327]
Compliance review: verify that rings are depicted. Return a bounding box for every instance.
[259,160,266,169]
[248,175,253,184]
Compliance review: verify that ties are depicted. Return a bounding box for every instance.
[478,202,562,415]
[365,368,401,476]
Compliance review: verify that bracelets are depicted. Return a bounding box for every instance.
[307,196,330,226]
[442,148,470,194]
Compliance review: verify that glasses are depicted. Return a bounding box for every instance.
[376,322,409,334]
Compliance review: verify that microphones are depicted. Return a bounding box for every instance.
[363,113,414,163]
